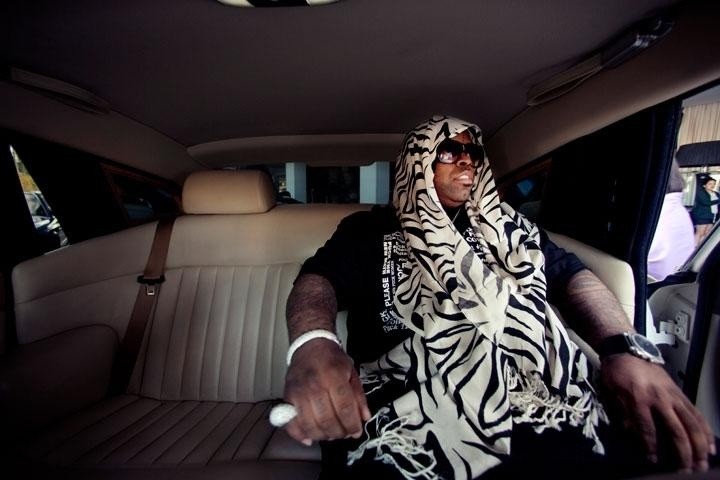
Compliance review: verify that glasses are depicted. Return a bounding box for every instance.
[436,138,484,168]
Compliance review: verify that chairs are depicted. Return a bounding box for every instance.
[0,167,635,479]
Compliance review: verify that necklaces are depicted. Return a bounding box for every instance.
[451,206,463,223]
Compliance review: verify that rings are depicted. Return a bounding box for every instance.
[270,402,299,428]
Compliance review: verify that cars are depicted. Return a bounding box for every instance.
[24,192,68,253]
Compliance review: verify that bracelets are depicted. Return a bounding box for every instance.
[285,329,342,367]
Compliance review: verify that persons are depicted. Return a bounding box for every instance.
[646,154,696,281]
[692,178,719,248]
[269,114,716,480]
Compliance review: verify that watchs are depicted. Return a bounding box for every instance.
[595,330,666,365]
